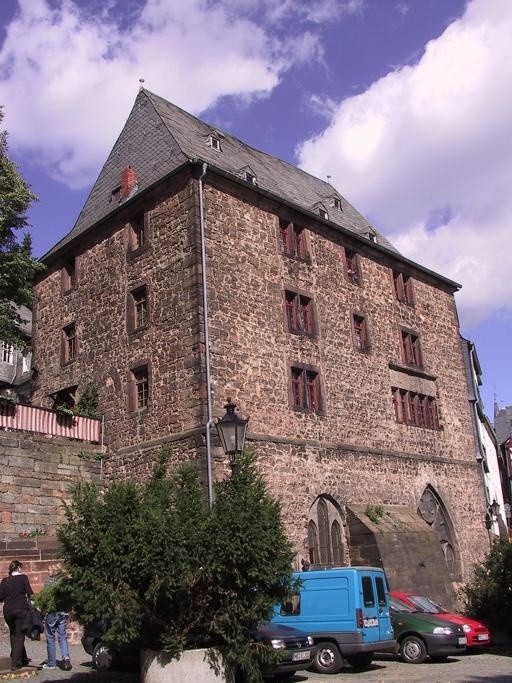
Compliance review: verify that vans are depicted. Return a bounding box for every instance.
[261,567,396,673]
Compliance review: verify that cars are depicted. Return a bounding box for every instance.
[81,612,314,683]
[386,591,489,663]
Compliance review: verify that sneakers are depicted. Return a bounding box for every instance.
[64,657,72,670]
[43,663,56,670]
[10,660,28,670]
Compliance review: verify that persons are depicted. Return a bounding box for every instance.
[37,563,72,670]
[0,560,34,671]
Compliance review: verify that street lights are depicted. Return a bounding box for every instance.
[215,398,251,479]
